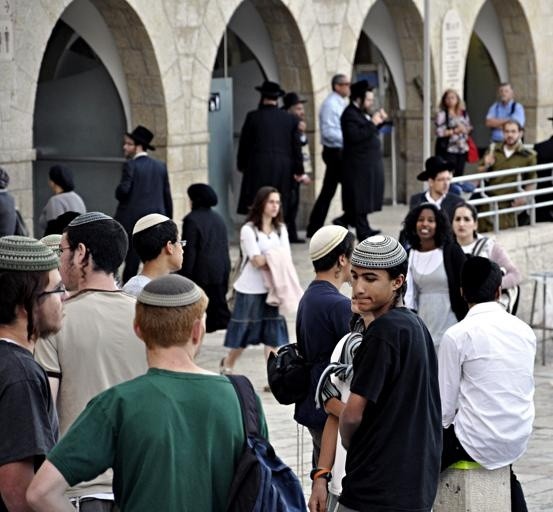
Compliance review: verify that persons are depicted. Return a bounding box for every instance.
[437,261,537,512]
[410,81,553,230]
[32,212,149,512]
[25,274,270,512]
[2,163,305,394]
[235,73,388,242]
[332,235,444,512]
[398,201,467,358]
[453,203,522,314]
[115,126,174,284]
[307,286,376,512]
[0,235,71,512]
[293,224,359,469]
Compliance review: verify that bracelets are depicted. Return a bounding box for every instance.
[310,468,332,481]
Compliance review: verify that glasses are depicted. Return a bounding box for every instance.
[177,240,185,246]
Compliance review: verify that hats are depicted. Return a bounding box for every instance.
[136,275,203,308]
[1,234,60,271]
[132,213,169,236]
[254,81,284,99]
[350,79,374,101]
[68,211,113,227]
[349,235,407,269]
[279,92,306,113]
[127,125,157,151]
[416,156,457,181]
[189,182,218,207]
[310,225,348,264]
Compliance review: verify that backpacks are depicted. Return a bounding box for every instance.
[226,222,259,313]
[315,329,365,408]
[226,374,308,512]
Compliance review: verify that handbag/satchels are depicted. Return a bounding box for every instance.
[266,342,311,406]
[496,267,520,316]
[467,136,478,164]
